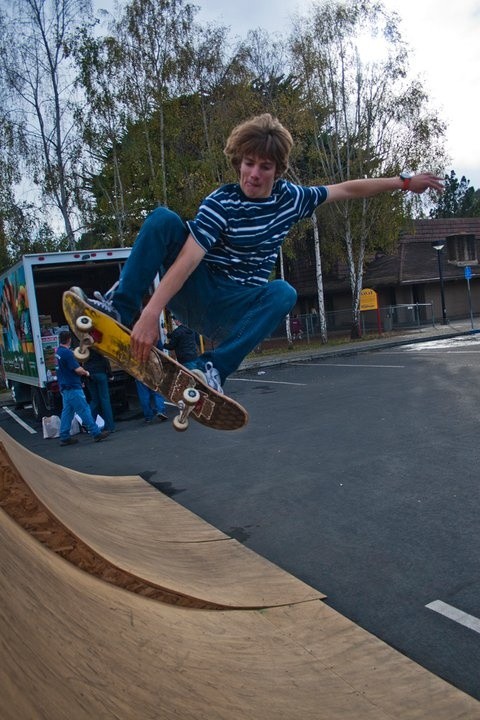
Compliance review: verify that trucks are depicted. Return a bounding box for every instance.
[0,247,171,420]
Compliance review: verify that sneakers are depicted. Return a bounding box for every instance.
[70,286,123,323]
[193,368,222,391]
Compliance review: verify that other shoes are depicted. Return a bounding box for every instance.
[60,438,79,446]
[107,427,122,432]
[145,420,151,422]
[156,413,168,420]
[81,425,89,435]
[94,430,110,441]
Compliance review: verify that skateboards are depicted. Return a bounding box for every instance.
[60,290,251,433]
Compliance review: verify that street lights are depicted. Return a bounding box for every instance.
[431,239,447,324]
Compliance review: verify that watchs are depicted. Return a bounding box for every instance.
[399,172,411,191]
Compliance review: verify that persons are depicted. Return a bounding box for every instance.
[71,113,447,398]
[54,331,111,446]
[84,351,122,433]
[136,341,169,423]
[291,314,303,341]
[164,314,203,370]
